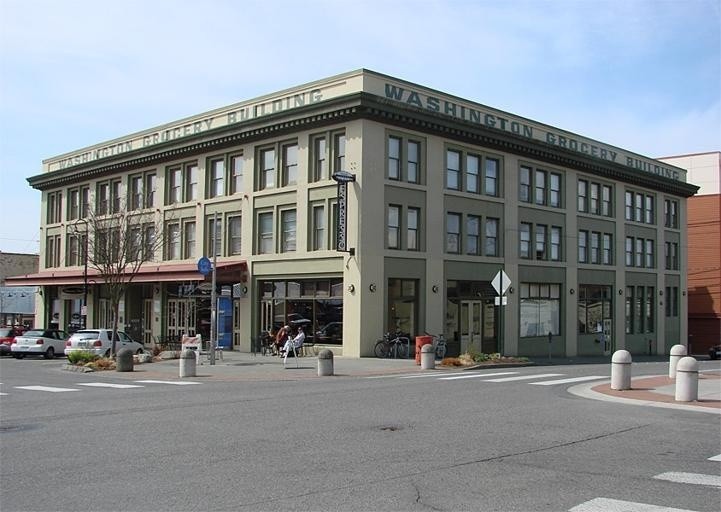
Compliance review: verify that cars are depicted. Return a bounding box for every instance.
[274,312,343,342]
[63,328,145,364]
[0,327,72,359]
[708,344,721,359]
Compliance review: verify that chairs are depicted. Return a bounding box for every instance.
[251,330,317,357]
[152,335,195,350]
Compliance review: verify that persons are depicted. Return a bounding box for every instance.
[6,326,32,358]
[268,325,306,358]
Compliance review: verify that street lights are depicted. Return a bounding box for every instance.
[75,218,88,330]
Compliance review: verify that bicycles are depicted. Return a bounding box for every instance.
[374,328,407,359]
[423,330,447,359]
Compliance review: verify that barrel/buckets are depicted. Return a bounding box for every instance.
[415,336,432,366]
